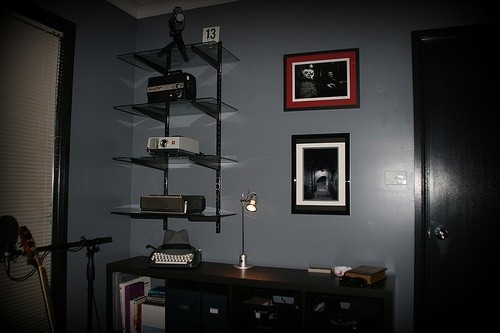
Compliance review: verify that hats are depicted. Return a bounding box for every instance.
[155,228,195,255]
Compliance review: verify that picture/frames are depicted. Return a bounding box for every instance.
[282,47,360,112]
[291,132,350,216]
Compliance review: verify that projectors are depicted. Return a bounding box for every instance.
[147,137,199,156]
[147,70,196,102]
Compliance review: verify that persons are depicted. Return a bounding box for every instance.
[326,72,339,88]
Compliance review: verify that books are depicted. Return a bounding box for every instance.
[307,266,331,274]
[243,293,299,333]
[118,276,164,333]
[345,265,388,285]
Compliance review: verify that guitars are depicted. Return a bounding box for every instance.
[17,226,59,333]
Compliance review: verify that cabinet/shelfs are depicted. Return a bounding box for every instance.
[109,6,240,233]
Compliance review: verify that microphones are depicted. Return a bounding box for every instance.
[0,215,19,271]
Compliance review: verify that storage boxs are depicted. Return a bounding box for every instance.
[167,288,228,328]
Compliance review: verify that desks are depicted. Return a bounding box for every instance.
[105,255,396,333]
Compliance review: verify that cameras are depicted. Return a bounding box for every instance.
[169,7,185,32]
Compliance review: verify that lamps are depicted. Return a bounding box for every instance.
[232,188,257,270]
